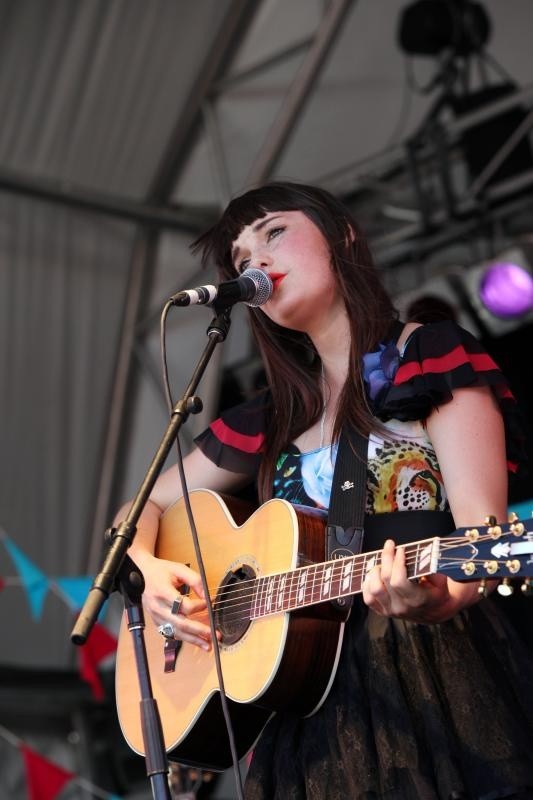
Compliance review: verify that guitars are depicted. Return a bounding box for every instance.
[112,488,533,771]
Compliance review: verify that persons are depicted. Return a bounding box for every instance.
[109,181,533,800]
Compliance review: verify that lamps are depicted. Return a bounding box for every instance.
[460,245,533,335]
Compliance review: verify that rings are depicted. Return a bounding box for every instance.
[171,596,183,615]
[158,624,174,638]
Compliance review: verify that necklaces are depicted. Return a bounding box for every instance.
[319,363,326,449]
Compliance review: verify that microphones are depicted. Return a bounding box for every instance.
[171,266,275,307]
